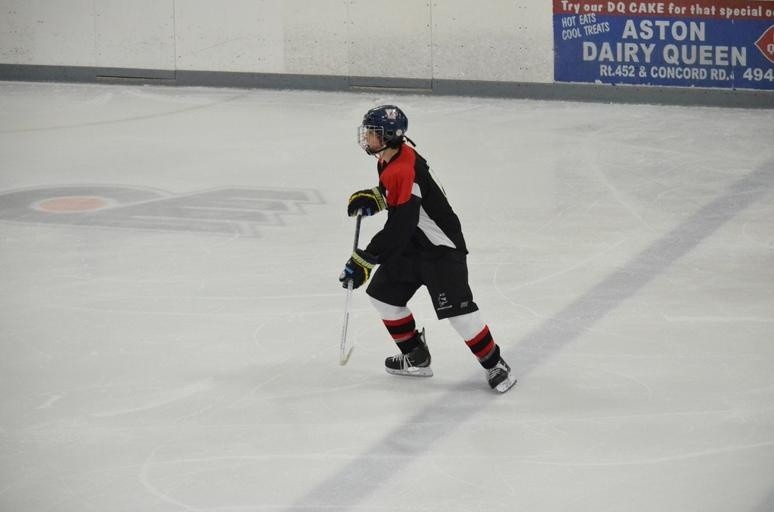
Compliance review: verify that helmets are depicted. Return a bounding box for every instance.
[359,105,407,155]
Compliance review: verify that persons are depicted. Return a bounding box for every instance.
[339,103,512,389]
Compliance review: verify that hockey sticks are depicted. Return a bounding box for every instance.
[340,208,363,365]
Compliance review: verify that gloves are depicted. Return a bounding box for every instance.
[340,249,376,289]
[348,186,387,216]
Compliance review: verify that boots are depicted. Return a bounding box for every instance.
[480,345,511,388]
[385,327,431,369]
